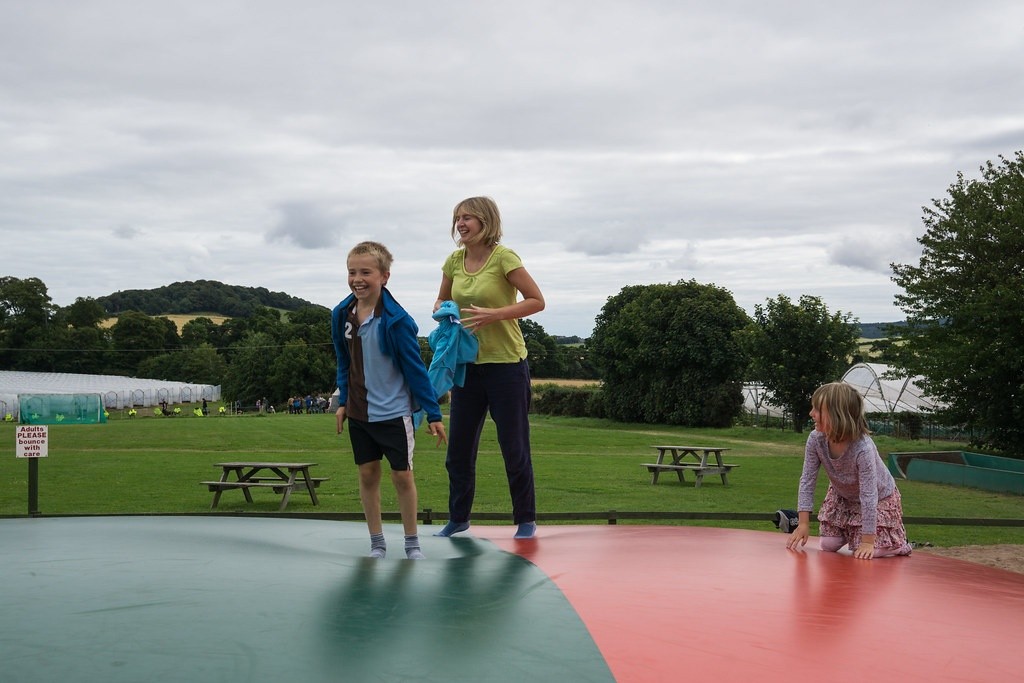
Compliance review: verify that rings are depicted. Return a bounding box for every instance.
[476,321,477,325]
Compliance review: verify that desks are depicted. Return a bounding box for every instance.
[649,445,729,489]
[210,461,319,511]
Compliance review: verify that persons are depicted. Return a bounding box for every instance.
[236,401,241,408]
[432,197,545,539]
[332,242,447,561]
[263,397,269,411]
[787,382,913,559]
[288,394,327,414]
[202,398,207,415]
[162,398,167,416]
[256,400,261,411]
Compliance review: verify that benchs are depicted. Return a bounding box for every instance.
[199,481,292,488]
[640,463,710,471]
[248,476,330,481]
[677,461,741,468]
